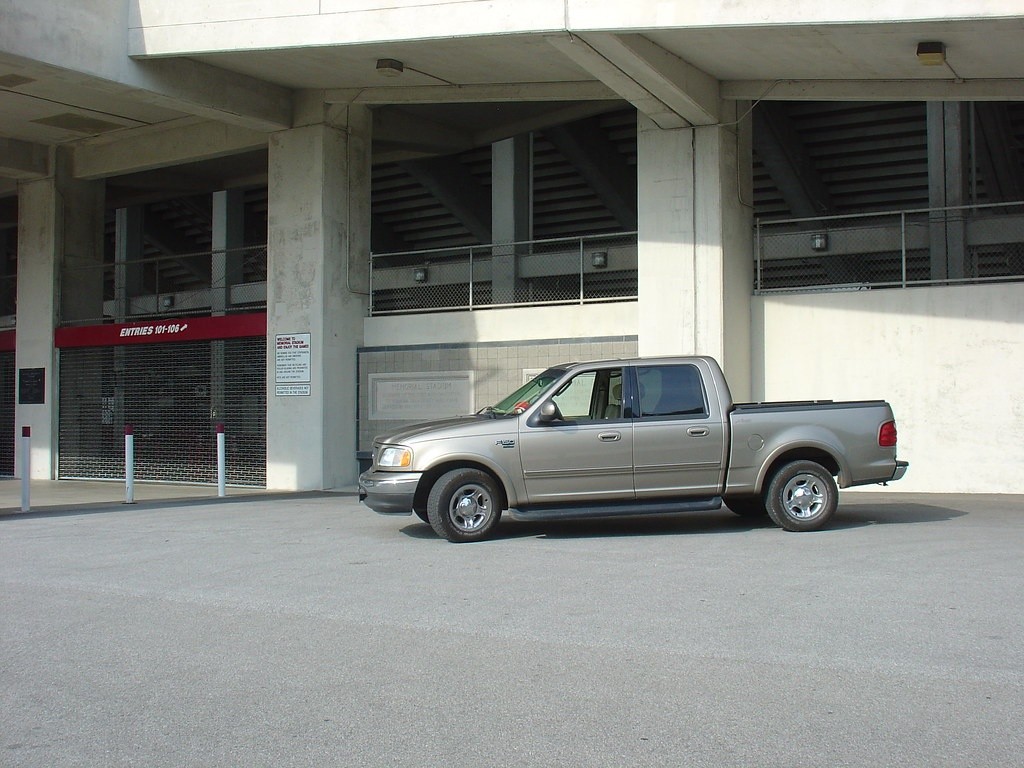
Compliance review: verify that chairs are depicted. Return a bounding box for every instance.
[605,384,626,419]
[624,381,645,418]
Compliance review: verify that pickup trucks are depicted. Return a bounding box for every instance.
[357,355,909,542]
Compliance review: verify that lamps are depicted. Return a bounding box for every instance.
[591,251,607,267]
[413,268,429,282]
[916,41,946,68]
[377,59,403,79]
[810,233,828,249]
[162,296,175,307]
